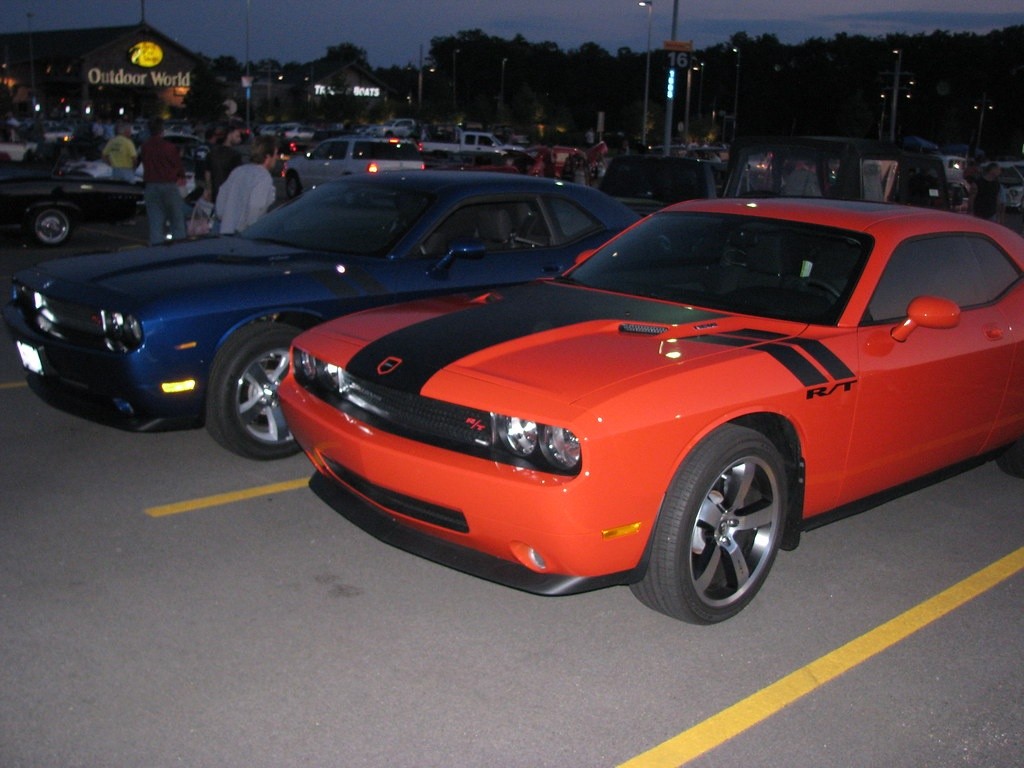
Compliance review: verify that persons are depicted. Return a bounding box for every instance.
[585,128,595,149]
[965,147,1007,223]
[91,117,113,140]
[139,117,187,244]
[204,127,242,215]
[688,138,710,148]
[116,112,129,130]
[192,130,217,182]
[102,122,138,185]
[75,113,104,149]
[618,138,630,155]
[710,150,722,162]
[214,137,280,234]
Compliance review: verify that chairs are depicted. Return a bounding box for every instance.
[473,205,515,251]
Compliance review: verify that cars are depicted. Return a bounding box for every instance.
[0,117,1023,249]
[0,169,648,462]
[271,198,1023,628]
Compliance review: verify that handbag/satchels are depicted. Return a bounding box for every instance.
[186,203,210,236]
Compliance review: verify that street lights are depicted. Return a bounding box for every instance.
[698,62,705,114]
[636,0,654,148]
[26,12,38,112]
[974,90,994,147]
[452,49,461,109]
[501,57,508,106]
[731,45,741,145]
[890,48,903,145]
